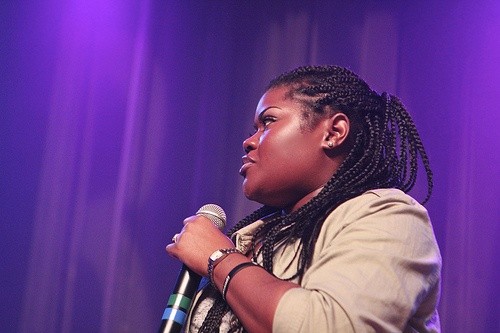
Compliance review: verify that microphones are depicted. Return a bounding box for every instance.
[155,204,227,333]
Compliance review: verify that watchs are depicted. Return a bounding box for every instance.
[207,248,246,291]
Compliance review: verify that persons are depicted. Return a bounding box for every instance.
[166,64,442,333]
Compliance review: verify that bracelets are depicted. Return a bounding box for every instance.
[221,262,264,305]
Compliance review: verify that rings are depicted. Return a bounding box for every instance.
[173,233,179,243]
